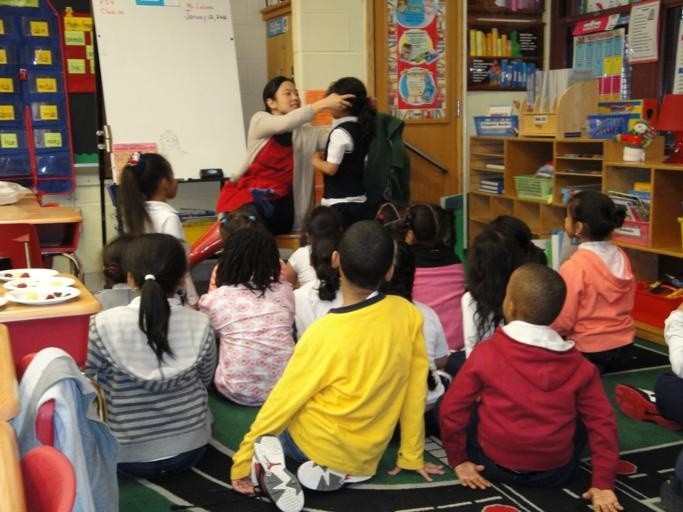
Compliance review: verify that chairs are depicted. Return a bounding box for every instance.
[18,446,77,508]
[41,223,83,277]
[19,353,54,444]
[1,223,43,271]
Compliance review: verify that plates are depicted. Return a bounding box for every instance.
[1,269,82,307]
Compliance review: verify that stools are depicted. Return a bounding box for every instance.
[273,229,304,253]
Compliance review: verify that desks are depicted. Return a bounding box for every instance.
[0,422,26,508]
[0,206,89,278]
[0,325,19,420]
[0,273,106,428]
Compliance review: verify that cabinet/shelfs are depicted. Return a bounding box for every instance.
[463,1,546,92]
[467,135,683,347]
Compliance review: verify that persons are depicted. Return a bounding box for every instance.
[231,222,447,512]
[439,263,624,511]
[195,202,549,442]
[215,76,377,223]
[614,304,683,434]
[122,154,202,308]
[88,234,217,479]
[91,235,136,313]
[313,77,372,227]
[557,190,637,373]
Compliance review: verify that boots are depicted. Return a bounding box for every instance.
[186,219,222,272]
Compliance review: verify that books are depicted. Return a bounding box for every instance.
[476,178,502,196]
[468,27,513,59]
[610,181,654,226]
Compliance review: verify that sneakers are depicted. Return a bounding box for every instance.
[297,459,373,491]
[254,434,305,512]
[615,384,683,430]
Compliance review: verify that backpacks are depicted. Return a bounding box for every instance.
[362,112,411,221]
